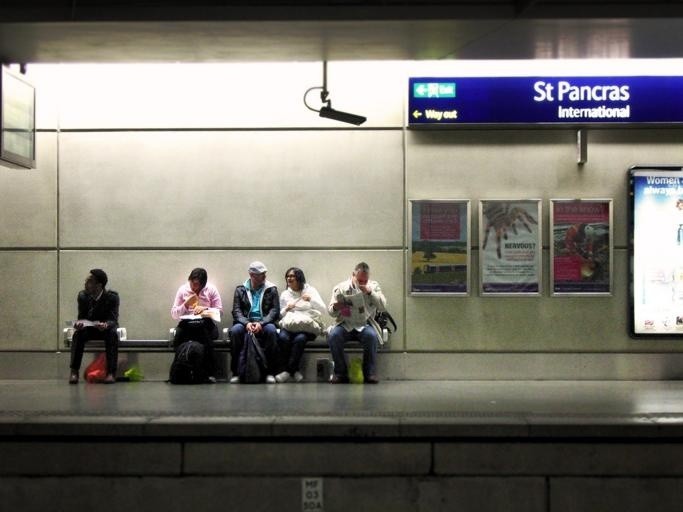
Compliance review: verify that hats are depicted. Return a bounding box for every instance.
[248,262,267,274]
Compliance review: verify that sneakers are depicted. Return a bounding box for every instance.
[365,374,379,384]
[105,375,115,382]
[209,377,217,384]
[230,376,238,384]
[267,372,304,384]
[69,368,79,384]
[328,376,348,382]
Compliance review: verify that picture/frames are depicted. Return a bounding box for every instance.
[407,199,472,297]
[549,198,614,298]
[478,199,542,297]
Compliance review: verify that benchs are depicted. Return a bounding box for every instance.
[63,328,388,381]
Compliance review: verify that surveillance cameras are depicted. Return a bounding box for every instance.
[319,107,366,126]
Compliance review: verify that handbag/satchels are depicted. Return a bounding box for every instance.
[85,354,106,383]
[376,308,396,333]
[121,365,143,381]
[348,355,365,384]
[281,309,323,335]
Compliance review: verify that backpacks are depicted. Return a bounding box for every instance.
[237,332,268,384]
[169,341,205,384]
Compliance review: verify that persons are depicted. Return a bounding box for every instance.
[171,268,224,384]
[676,199,683,245]
[229,261,280,383]
[327,262,386,384]
[275,267,326,383]
[68,269,121,384]
